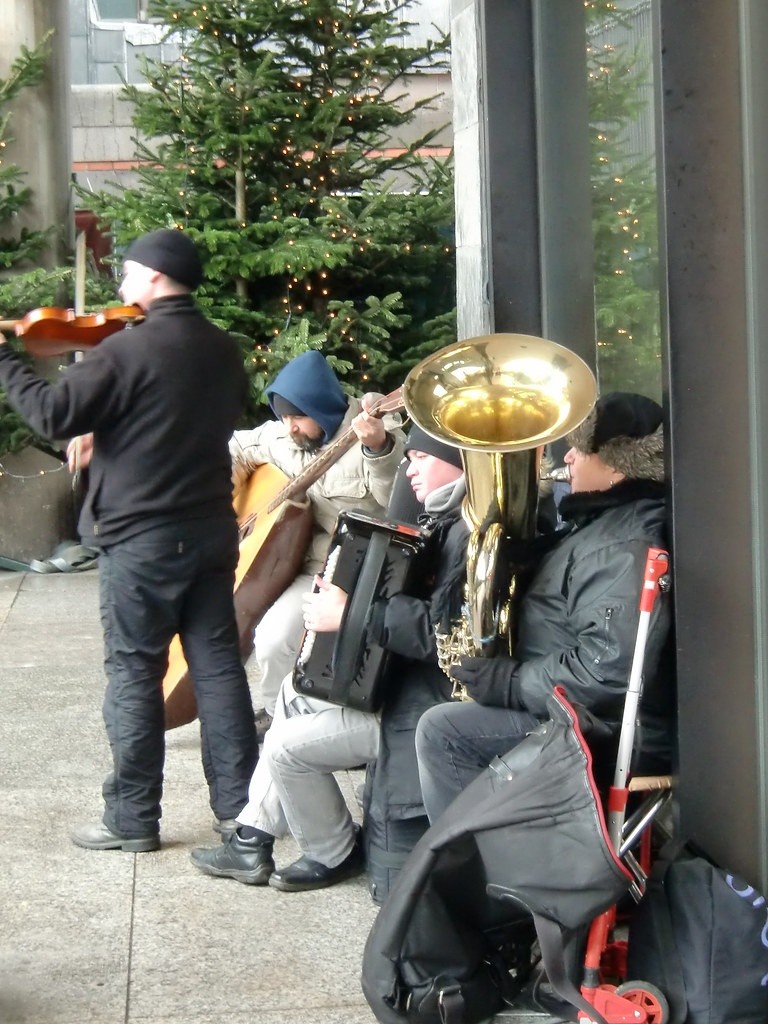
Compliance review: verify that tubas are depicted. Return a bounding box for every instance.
[401,332,599,704]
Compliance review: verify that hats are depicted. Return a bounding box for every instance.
[566,391,666,485]
[272,392,309,416]
[403,421,466,470]
[123,227,203,290]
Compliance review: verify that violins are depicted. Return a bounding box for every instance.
[0,306,146,356]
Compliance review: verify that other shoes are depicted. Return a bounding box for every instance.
[269,822,364,892]
[211,818,237,843]
[69,820,162,853]
[190,828,277,886]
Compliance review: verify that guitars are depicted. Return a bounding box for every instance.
[163,384,405,732]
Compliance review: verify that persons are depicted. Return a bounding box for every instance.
[189,424,471,891]
[0,229,259,853]
[413,392,679,825]
[228,350,406,743]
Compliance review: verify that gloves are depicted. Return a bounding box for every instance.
[450,641,522,712]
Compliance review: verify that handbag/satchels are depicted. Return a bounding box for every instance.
[631,824,768,1023]
